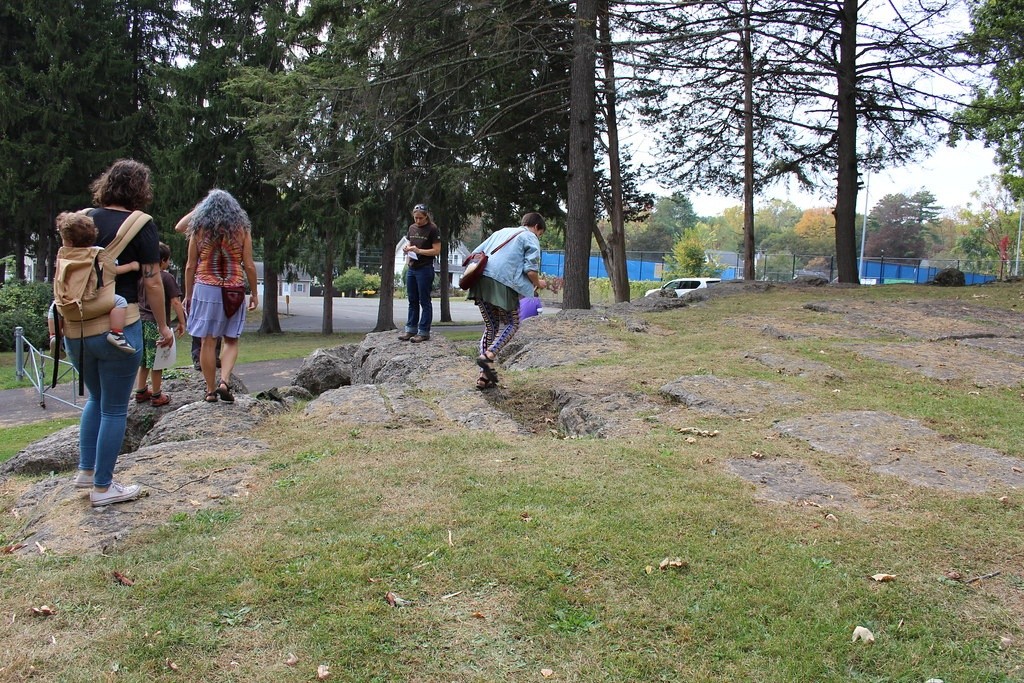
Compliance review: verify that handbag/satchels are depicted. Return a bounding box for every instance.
[221,286,245,319]
[459,250,489,290]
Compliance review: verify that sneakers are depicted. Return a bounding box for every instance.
[74,473,94,487]
[90,481,141,506]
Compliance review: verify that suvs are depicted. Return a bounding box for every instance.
[643,277,721,299]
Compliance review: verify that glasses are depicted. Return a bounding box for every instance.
[414,206,427,211]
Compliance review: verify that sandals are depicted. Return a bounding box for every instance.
[204,391,217,402]
[216,380,234,404]
[476,377,497,391]
[476,353,499,383]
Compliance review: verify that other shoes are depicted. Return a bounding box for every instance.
[49,336,66,359]
[136,386,151,403]
[150,390,171,407]
[216,358,221,367]
[398,332,417,340]
[194,361,201,370]
[106,330,136,353]
[410,335,430,342]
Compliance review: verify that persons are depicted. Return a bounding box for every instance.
[184,189,258,402]
[63,159,174,506]
[48,214,137,360]
[519,291,543,322]
[465,212,547,389]
[136,242,186,407]
[175,211,222,370]
[398,204,441,343]
